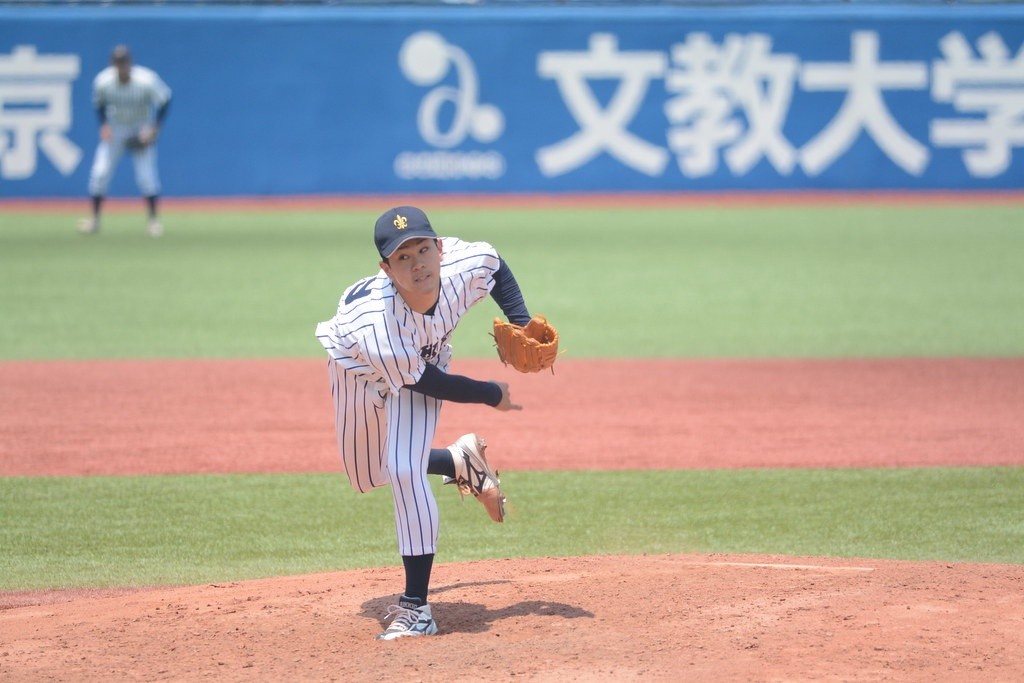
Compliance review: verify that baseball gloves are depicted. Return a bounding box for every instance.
[487,312,561,377]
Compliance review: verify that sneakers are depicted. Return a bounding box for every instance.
[441,433,506,523]
[376,595,438,640]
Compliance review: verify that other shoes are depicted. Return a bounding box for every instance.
[149,223,161,237]
[82,220,101,235]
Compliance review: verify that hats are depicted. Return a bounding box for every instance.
[375,206,438,258]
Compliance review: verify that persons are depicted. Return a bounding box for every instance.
[79,43,174,239]
[315,204,558,639]
